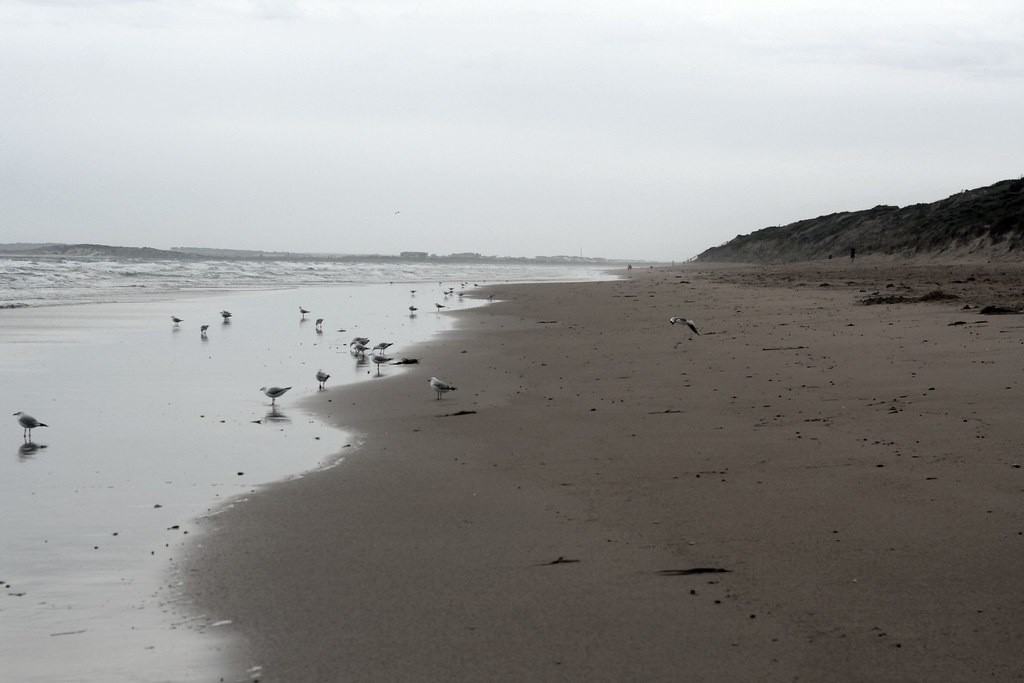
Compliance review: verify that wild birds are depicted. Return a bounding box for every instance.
[406,278,497,317]
[259,386,291,406]
[670,317,698,335]
[169,311,232,340]
[299,306,312,318]
[350,337,393,377]
[315,318,323,329]
[316,369,330,391]
[15,411,47,440]
[19,444,46,460]
[428,377,456,401]
